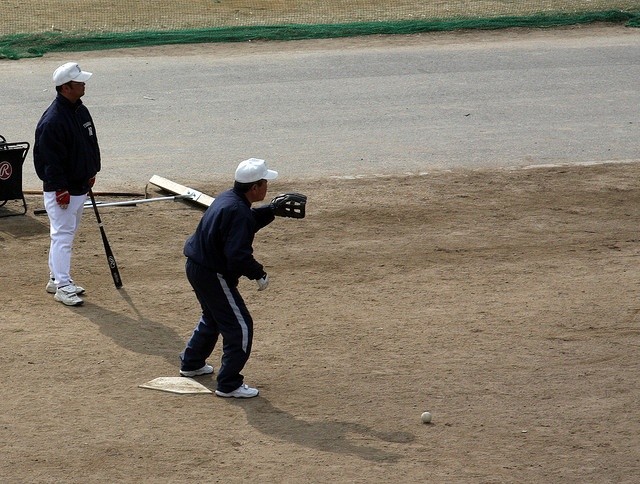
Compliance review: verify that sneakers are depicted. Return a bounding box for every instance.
[45,277,85,295]
[179,363,214,376]
[216,381,258,398]
[54,283,84,306]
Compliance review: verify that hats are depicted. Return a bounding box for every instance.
[53,61,92,86]
[235,157,279,183]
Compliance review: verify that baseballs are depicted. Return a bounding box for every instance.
[421,412,432,422]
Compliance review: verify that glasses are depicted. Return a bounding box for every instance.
[70,81,84,84]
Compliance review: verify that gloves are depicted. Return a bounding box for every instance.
[55,188,70,209]
[89,175,95,186]
[256,271,269,290]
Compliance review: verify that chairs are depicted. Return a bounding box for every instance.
[0,134,30,218]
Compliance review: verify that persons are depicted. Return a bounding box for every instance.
[33,62,101,306]
[178,158,286,398]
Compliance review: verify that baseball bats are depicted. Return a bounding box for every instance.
[89,189,122,289]
[34,202,101,215]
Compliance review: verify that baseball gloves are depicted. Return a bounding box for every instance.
[270,193,307,219]
